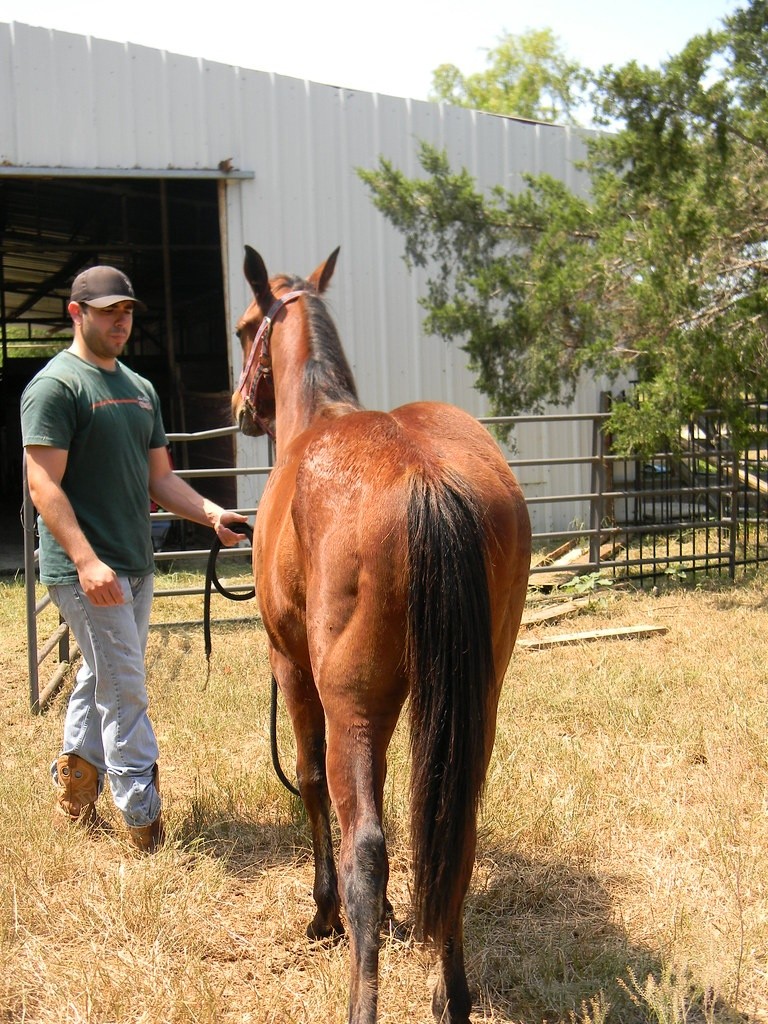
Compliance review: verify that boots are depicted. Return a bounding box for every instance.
[126,762,165,854]
[55,754,118,842]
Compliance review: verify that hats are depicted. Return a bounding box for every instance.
[71,266,147,310]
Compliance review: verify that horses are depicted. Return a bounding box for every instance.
[231,245,532,1024]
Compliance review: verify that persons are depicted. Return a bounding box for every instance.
[15,265,250,861]
